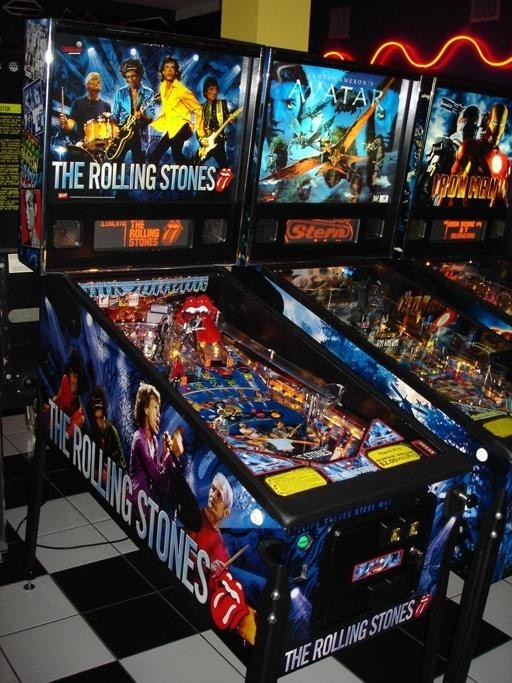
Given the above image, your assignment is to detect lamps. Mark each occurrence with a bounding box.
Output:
[221,1,312,54]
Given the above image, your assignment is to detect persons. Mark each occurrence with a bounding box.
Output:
[87,385,127,489]
[59,71,112,148]
[39,350,86,448]
[99,59,156,196]
[175,471,232,592]
[141,57,203,195]
[124,380,186,534]
[263,62,312,150]
[133,311,331,456]
[194,75,240,195]
